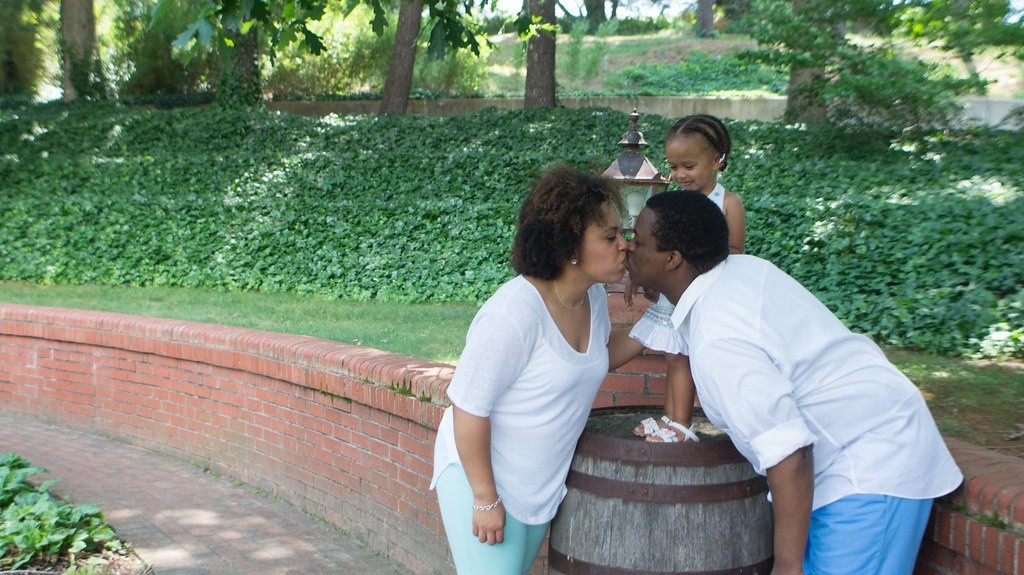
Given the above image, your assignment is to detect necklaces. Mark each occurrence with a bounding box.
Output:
[552,278,584,309]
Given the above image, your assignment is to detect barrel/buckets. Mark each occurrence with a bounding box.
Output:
[548,404,774,575]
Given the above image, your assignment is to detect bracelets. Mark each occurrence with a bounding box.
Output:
[471,495,502,512]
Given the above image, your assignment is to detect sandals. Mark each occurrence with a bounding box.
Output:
[646,421,700,443]
[633,413,671,436]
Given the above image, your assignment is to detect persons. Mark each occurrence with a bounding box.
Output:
[624,115,746,444]
[432,166,647,575]
[624,191,965,575]
